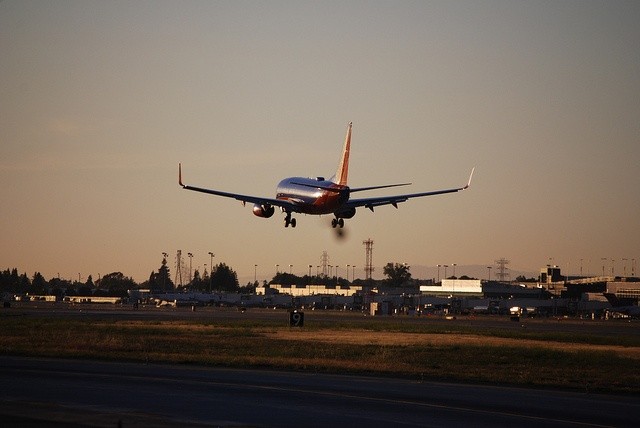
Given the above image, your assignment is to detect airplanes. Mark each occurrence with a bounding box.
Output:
[179,120,475,228]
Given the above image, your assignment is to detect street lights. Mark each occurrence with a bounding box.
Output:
[452,263,456,278]
[437,264,441,283]
[254,264,355,282]
[187,253,194,289]
[208,251,214,291]
[444,264,448,279]
